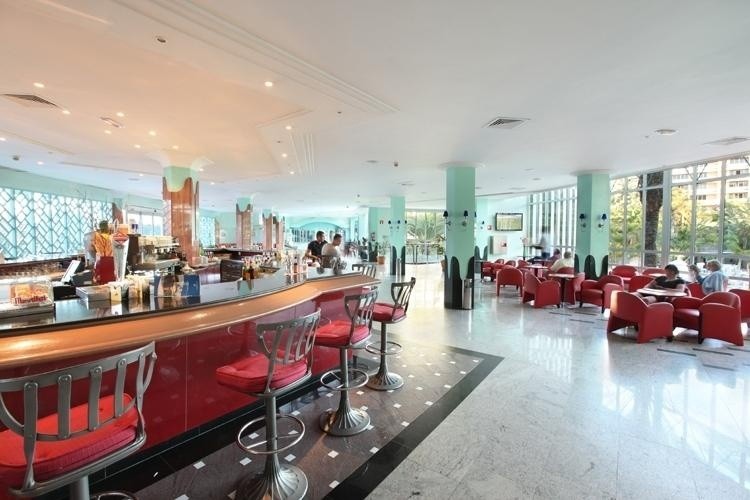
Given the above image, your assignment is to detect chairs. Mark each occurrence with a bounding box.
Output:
[0,342,160,500]
[480,259,750,347]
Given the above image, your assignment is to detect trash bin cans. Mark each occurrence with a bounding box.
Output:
[397,258,401,276]
[463,278,473,310]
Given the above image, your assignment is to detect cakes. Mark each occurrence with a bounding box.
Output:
[10,283,50,307]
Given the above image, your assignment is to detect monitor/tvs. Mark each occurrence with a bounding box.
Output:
[495,212,523,231]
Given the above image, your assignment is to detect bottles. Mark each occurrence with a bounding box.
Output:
[199,250,214,265]
[156,246,176,257]
[245,249,308,281]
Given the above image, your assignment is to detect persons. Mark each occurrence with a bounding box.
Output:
[688,265,704,283]
[531,225,551,258]
[630,264,685,304]
[548,247,561,265]
[699,259,732,296]
[321,233,344,259]
[550,251,574,273]
[306,230,329,261]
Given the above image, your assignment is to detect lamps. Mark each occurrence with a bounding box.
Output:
[442,211,451,226]
[579,213,586,228]
[462,210,469,226]
[598,213,607,228]
